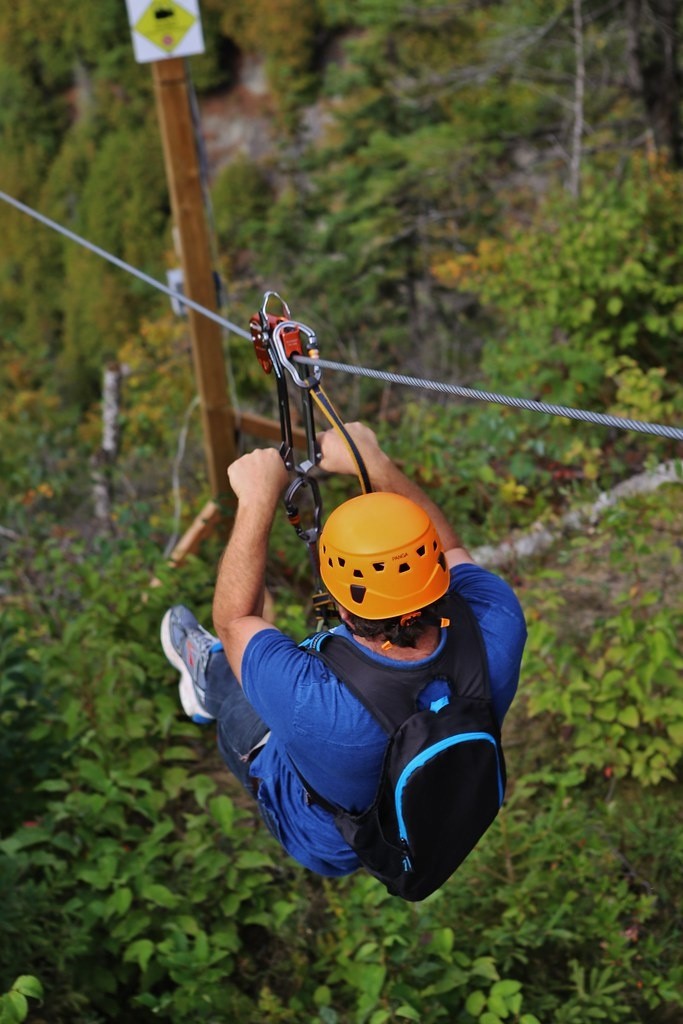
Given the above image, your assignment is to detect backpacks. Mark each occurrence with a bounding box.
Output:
[289,593,508,903]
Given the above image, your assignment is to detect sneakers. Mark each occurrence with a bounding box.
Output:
[161,606,223,725]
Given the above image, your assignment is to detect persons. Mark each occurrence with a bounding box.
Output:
[160,422,528,877]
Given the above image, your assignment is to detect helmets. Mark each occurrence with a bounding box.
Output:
[318,492,450,620]
